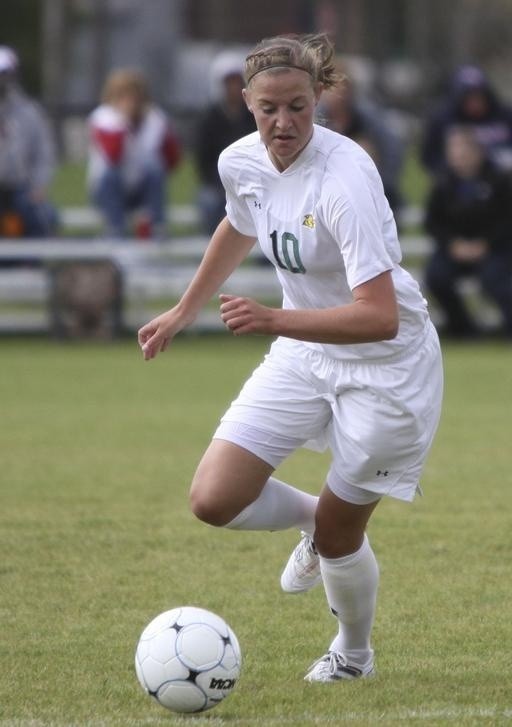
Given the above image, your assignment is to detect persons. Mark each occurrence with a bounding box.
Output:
[86,64,183,244]
[195,46,257,236]
[137,28,445,684]
[1,46,61,268]
[314,56,407,238]
[419,66,511,344]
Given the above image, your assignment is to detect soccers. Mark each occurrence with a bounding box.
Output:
[134,607,242,713]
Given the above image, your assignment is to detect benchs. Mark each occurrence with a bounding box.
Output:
[3,202,501,339]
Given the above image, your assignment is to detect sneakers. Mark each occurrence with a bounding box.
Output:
[304,639,375,685]
[281,534,322,593]
